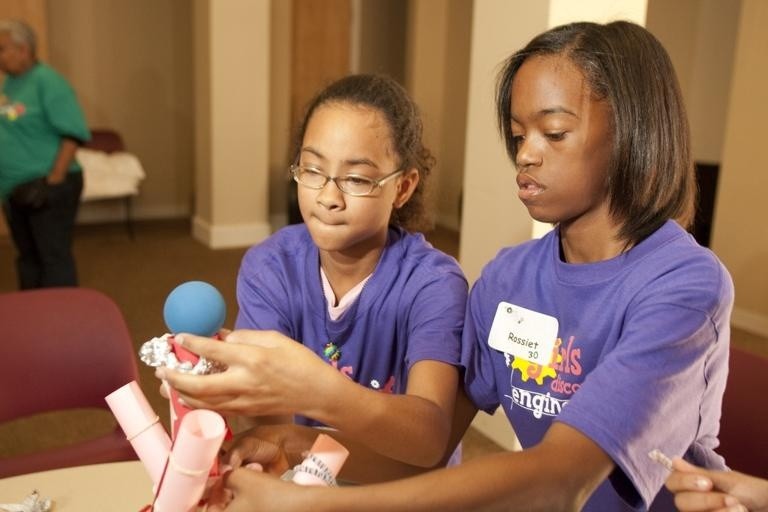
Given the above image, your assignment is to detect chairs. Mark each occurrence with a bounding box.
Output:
[0,286,151,478]
[71,131,134,243]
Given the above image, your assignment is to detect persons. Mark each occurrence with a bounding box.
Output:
[0,19,91,290]
[155,73,468,468]
[205,20,733,512]
[665,455,768,512]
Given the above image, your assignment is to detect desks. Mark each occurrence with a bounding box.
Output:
[5,460,369,512]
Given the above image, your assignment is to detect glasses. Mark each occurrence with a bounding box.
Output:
[290,152,404,196]
